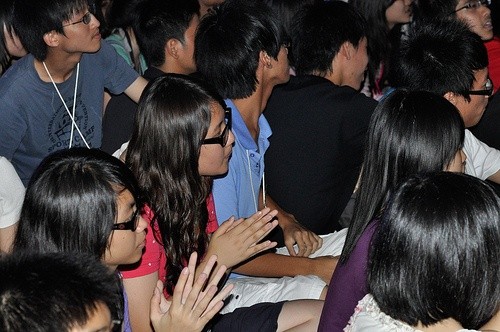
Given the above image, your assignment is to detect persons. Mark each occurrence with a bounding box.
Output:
[260,0,500,332]
[0,0,349,332]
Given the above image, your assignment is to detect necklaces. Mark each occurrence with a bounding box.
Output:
[43,61,90,149]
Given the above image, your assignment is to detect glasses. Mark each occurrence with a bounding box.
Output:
[62,3,96,27]
[205,107,232,148]
[468,77,493,96]
[114,207,142,232]
[456,0,492,12]
